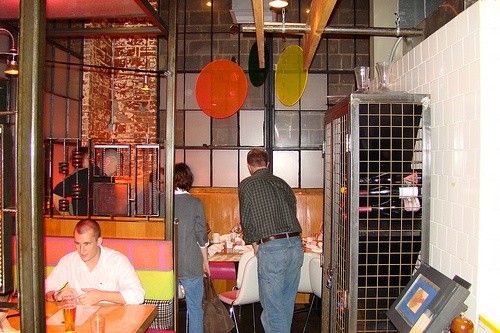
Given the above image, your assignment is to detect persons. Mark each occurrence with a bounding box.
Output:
[120,167,164,215]
[160,162,211,333]
[45,219,145,305]
[238,148,304,333]
[53,147,123,216]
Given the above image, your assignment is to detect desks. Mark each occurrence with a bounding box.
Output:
[209,252,242,262]
[0,298,159,333]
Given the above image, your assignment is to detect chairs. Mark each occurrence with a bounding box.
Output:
[290,252,323,333]
[219,251,260,333]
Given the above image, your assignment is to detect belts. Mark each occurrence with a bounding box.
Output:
[257,232,300,245]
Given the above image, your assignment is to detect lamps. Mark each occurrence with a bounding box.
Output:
[268,0,289,8]
[0,28,19,75]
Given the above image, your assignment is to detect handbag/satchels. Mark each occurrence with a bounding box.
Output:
[203,271,235,333]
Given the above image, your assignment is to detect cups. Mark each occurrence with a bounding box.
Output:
[62,295,77,333]
[354,66,371,94]
[89,312,106,333]
[374,62,393,93]
[230,233,237,242]
[211,232,220,243]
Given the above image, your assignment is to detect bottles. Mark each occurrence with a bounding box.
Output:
[337,171,422,220]
[450,311,474,333]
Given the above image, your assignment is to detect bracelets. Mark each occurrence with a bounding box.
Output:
[52,294,56,300]
